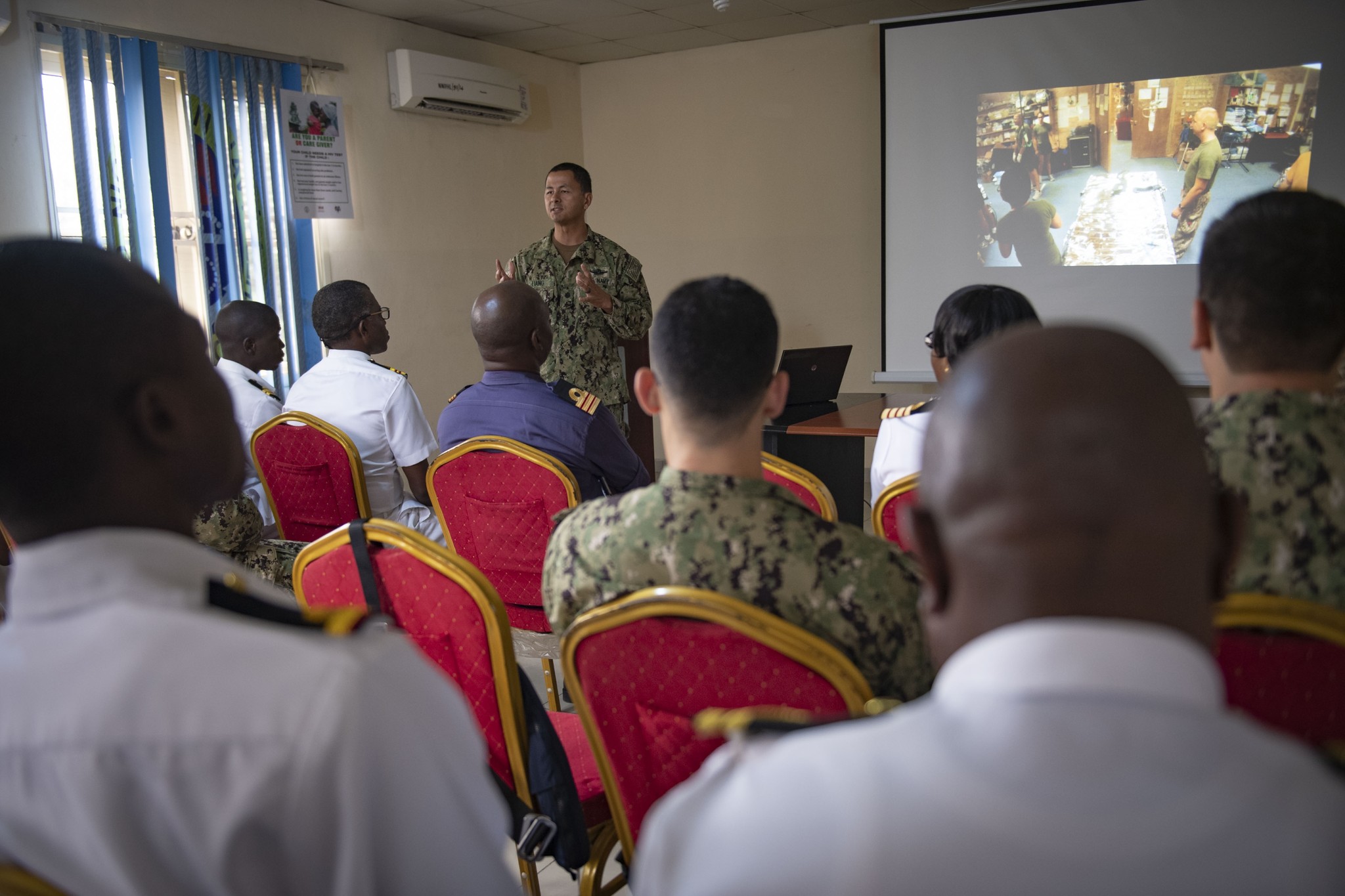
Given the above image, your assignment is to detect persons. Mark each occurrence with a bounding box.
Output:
[0,239,522,896]
[629,331,1345,896]
[495,163,653,440]
[975,111,1062,267]
[1171,107,1222,263]
[215,188,1345,700]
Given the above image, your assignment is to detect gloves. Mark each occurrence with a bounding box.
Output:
[1013,152,1022,163]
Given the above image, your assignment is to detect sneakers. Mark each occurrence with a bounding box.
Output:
[1049,175,1055,181]
[992,232,997,240]
[979,237,994,248]
[1039,177,1042,183]
[1032,185,1036,191]
[1032,190,1042,200]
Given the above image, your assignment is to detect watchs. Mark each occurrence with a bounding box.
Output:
[1179,203,1185,210]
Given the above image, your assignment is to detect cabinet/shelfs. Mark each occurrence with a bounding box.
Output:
[975,97,1056,148]
[1217,84,1264,126]
[1069,136,1092,168]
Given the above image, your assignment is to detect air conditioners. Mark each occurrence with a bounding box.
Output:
[386,48,530,124]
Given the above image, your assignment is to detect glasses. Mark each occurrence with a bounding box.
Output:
[997,186,1003,192]
[367,307,390,320]
[924,331,934,348]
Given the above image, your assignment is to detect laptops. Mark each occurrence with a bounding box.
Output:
[777,344,853,405]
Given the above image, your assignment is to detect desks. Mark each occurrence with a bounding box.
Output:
[1246,131,1289,164]
[753,392,938,533]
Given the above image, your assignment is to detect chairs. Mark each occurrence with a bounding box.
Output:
[248,411,1345,895]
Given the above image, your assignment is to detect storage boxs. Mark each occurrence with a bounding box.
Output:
[1177,141,1249,171]
[982,135,1017,183]
